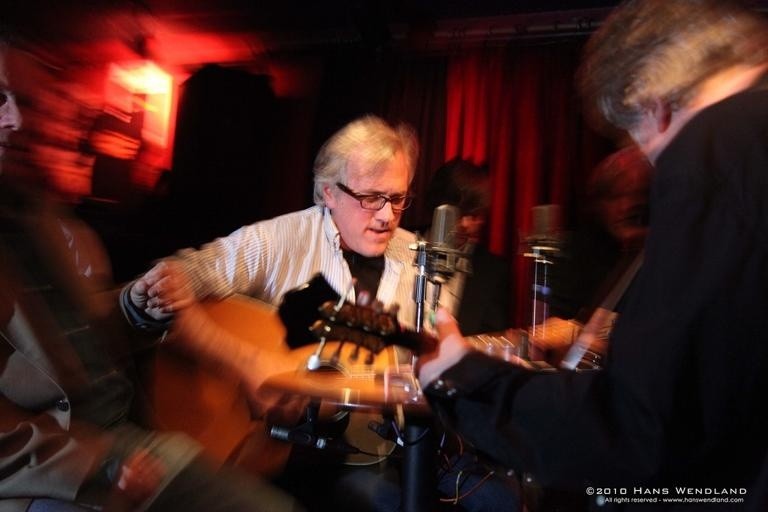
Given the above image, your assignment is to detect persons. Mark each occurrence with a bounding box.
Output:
[1,0,768,512]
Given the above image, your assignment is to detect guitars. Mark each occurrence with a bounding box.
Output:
[138,293,581,479]
[276,272,608,511]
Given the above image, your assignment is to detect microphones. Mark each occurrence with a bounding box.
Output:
[524,202,563,265]
[426,202,473,284]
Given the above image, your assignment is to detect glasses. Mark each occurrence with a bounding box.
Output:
[336,182,412,212]
